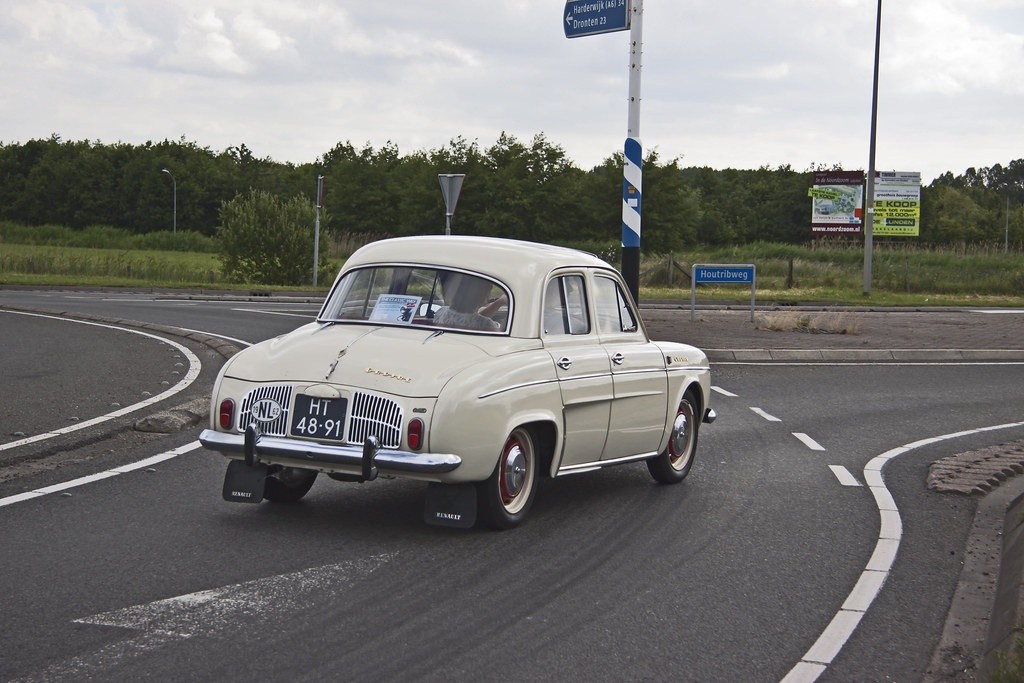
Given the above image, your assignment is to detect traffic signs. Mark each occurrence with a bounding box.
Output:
[563,0,631,38]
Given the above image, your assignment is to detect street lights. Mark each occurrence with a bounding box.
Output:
[162,168,177,233]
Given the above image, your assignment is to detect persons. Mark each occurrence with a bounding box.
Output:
[542,277,586,335]
[433,269,509,332]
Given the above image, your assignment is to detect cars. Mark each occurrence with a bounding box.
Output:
[200,235,716,535]
